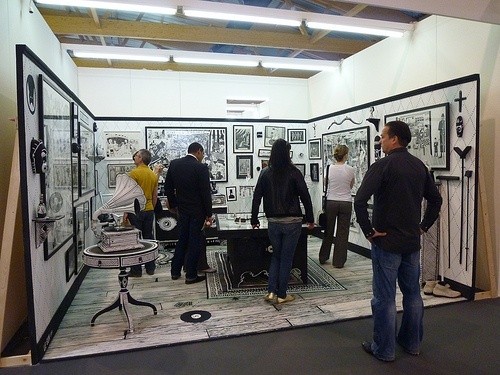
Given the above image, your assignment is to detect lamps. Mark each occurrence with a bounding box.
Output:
[29,0,414,72]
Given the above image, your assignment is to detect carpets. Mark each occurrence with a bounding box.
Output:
[205,246,347,296]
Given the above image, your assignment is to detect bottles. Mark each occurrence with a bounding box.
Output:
[38,194,46,218]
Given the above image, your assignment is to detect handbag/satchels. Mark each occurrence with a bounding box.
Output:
[319,213,327,226]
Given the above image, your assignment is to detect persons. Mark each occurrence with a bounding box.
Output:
[318,145,355,269]
[123,142,217,284]
[354,121,443,363]
[250,138,315,303]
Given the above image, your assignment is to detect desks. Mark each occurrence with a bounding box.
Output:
[82,241,160,332]
[216,213,321,285]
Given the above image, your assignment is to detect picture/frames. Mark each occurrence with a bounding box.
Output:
[383,102,450,169]
[309,163,318,181]
[287,128,306,144]
[294,163,306,177]
[103,130,143,160]
[239,184,254,198]
[321,125,372,198]
[108,163,139,190]
[264,126,286,147]
[350,210,360,232]
[236,155,253,178]
[38,74,99,282]
[226,187,237,201]
[232,125,255,153]
[308,138,321,160]
[145,126,228,181]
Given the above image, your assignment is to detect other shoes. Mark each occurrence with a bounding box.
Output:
[171,272,182,280]
[185,276,206,285]
[127,269,142,277]
[197,268,217,274]
[362,338,376,357]
[263,292,274,300]
[145,269,155,275]
[278,295,295,303]
